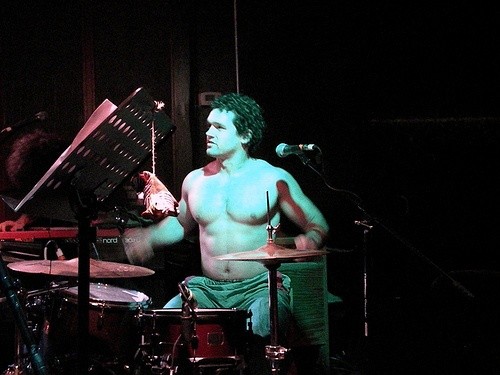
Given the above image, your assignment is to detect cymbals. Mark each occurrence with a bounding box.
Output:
[7,257,155,279]
[211,244,331,265]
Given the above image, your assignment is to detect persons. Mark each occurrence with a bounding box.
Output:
[0,129,62,232]
[123,92,330,337]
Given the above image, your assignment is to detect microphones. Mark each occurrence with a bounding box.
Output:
[276,143,317,158]
[0,111,49,135]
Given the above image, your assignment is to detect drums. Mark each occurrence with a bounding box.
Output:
[134,309,252,369]
[46,282,153,375]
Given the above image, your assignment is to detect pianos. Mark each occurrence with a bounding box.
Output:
[0,227,121,248]
